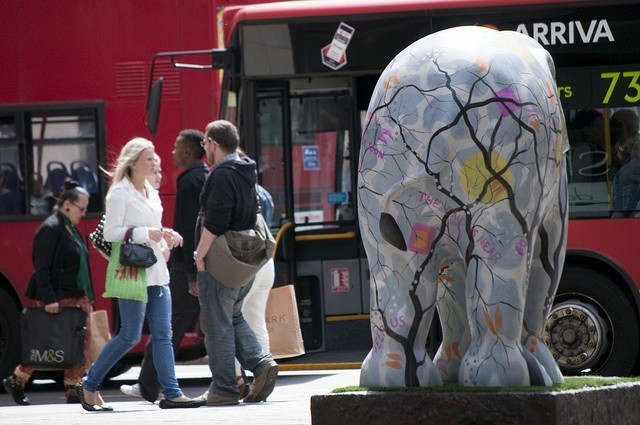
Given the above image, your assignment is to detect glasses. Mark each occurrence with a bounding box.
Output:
[70,201,87,213]
[200,139,221,148]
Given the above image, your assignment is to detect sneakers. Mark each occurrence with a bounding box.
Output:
[158,397,206,409]
[79,386,103,411]
[3,374,31,405]
[193,389,239,406]
[120,383,164,399]
[243,360,279,402]
[234,375,250,401]
[63,384,81,403]
[100,403,114,411]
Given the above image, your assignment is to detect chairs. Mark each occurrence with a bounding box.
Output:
[47,160,70,194]
[0,162,24,217]
[335,202,356,222]
[71,160,99,194]
[571,142,606,182]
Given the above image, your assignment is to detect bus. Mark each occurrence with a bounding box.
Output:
[146,1,640,376]
[1,1,384,395]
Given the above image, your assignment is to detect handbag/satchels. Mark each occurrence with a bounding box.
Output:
[194,211,276,288]
[119,228,157,267]
[88,214,112,261]
[18,307,87,370]
[264,284,307,360]
[101,242,149,303]
[83,309,111,367]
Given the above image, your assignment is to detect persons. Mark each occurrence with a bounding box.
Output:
[120,131,211,402]
[74,137,206,412]
[3,178,95,405]
[222,149,276,398]
[189,120,278,406]
[88,153,170,411]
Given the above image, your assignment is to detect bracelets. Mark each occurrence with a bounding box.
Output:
[191,251,207,261]
